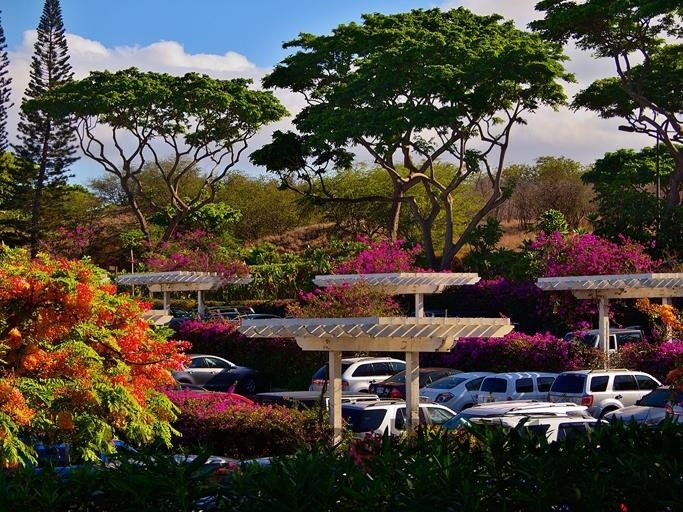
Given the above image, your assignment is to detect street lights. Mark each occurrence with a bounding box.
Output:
[615,124,662,234]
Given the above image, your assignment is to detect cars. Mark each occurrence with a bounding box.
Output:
[166,381,206,393]
[169,353,269,394]
[154,302,285,322]
[15,437,296,509]
[564,322,646,354]
